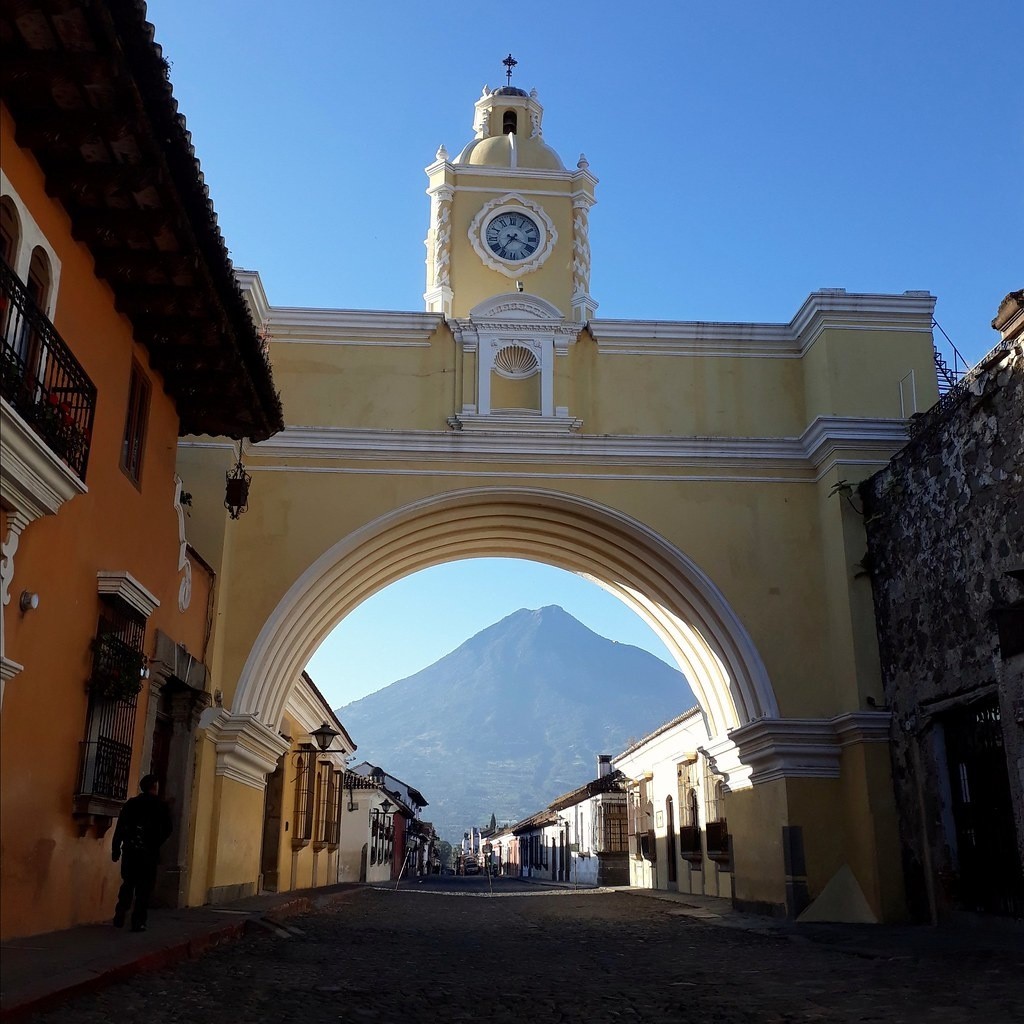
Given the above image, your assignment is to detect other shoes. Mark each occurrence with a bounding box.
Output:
[113,907,125,928]
[131,920,147,932]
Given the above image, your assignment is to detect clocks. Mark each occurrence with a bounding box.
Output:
[480,204,547,265]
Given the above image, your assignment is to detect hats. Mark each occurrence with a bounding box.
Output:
[140,775,161,791]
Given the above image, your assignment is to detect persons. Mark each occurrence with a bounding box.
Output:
[112,775,172,931]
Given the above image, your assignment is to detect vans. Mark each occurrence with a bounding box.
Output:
[465,862,479,874]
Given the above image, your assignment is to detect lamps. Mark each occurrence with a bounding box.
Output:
[290,721,346,783]
[369,798,395,829]
[828,479,863,515]
[224,440,251,520]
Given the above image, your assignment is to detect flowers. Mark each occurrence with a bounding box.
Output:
[0,352,91,468]
